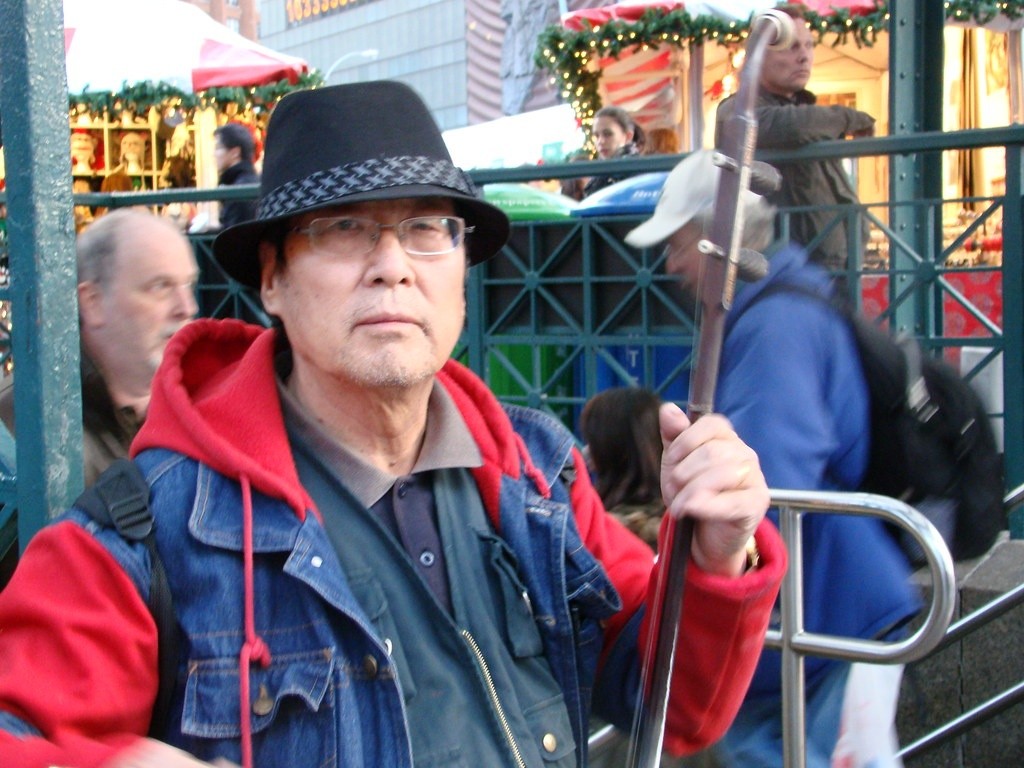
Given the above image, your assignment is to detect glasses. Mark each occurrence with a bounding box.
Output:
[287,216,477,258]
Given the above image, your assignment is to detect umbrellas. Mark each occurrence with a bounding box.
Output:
[65,1,315,94]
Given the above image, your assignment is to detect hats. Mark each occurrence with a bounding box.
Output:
[211,79,511,288]
[623,149,761,248]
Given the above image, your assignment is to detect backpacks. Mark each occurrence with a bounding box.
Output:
[741,273,1004,575]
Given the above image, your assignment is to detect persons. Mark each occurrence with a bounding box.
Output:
[712,6,877,317]
[0,206,204,595]
[1,77,791,767]
[156,155,196,231]
[579,108,649,198]
[626,143,920,767]
[211,122,266,230]
[98,173,134,197]
[576,387,738,768]
